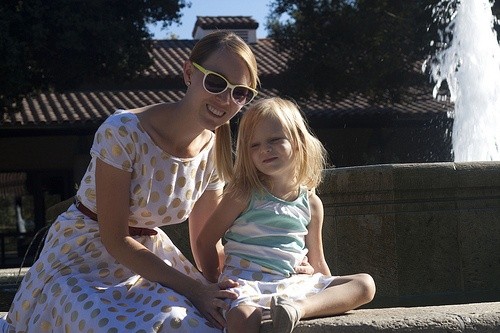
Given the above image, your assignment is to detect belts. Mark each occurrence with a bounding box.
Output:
[74,196,158,236]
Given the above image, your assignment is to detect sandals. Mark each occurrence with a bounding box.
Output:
[271,294,299,333]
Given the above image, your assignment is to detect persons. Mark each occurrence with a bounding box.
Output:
[197,97,376,333]
[2,31,259,332]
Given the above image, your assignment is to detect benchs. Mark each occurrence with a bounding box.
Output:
[46,163,500,333]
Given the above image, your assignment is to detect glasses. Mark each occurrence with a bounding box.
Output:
[193,62,258,106]
